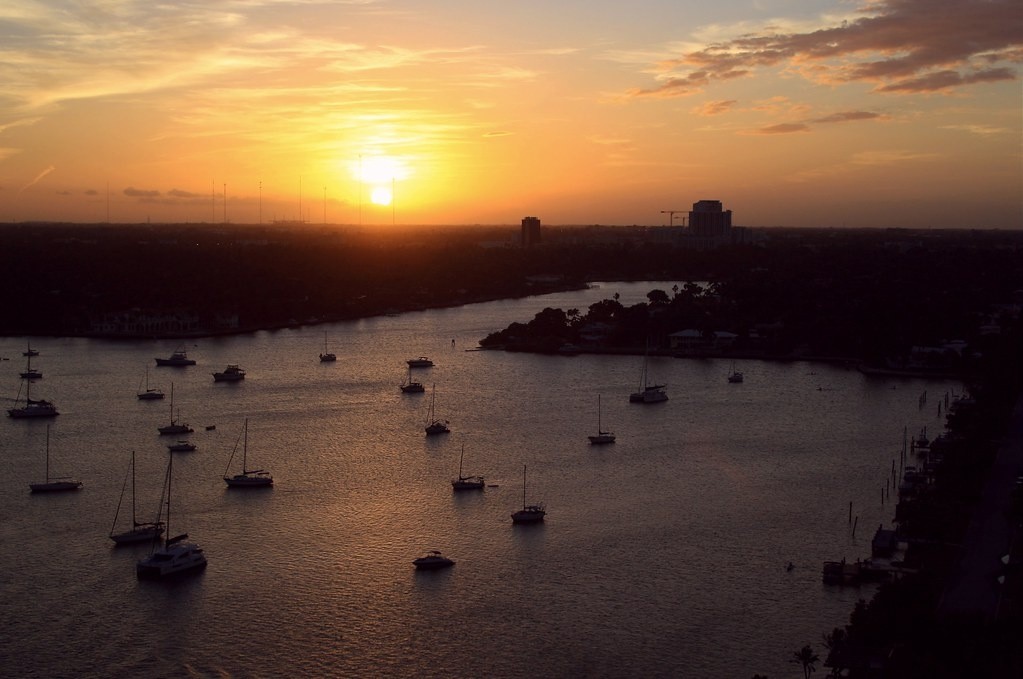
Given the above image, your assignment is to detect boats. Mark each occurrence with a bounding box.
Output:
[152,340,197,367]
[165,439,197,452]
[211,363,247,382]
[22,351,40,356]
[18,372,43,379]
[406,357,435,368]
[726,359,745,383]
[411,549,457,571]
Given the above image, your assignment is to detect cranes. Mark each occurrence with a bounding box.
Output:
[659,210,690,228]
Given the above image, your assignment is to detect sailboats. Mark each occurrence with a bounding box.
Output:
[5,340,61,420]
[318,331,337,361]
[109,448,166,547]
[588,395,616,444]
[136,451,208,585]
[157,382,195,436]
[510,465,547,523]
[451,440,486,491]
[27,424,84,493]
[630,332,669,403]
[400,366,426,393]
[136,363,165,399]
[222,417,275,489]
